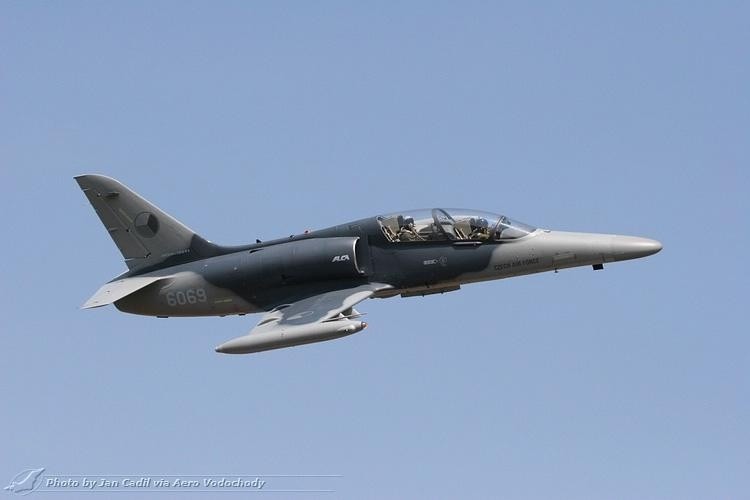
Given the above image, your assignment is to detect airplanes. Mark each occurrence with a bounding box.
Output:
[70,171,665,357]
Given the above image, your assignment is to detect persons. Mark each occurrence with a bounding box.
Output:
[400,216,420,241]
[472,218,490,239]
